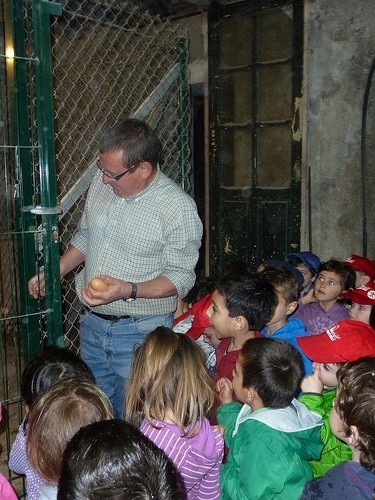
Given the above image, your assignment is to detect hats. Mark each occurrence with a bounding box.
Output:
[296,321,375,363]
[342,255,375,274]
[286,251,320,270]
[341,283,375,305]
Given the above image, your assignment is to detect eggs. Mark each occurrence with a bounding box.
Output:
[86,277,108,299]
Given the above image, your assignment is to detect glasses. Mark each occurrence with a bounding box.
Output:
[96,158,139,180]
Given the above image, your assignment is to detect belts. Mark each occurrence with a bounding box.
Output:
[84,305,130,320]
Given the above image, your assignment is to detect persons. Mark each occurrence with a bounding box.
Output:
[0,251,375,500]
[28,118,203,421]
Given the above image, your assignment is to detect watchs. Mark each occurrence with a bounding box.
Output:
[123,282,136,303]
